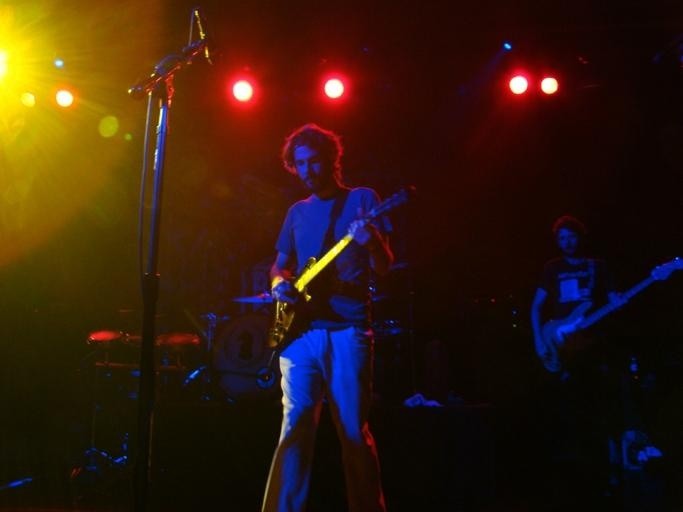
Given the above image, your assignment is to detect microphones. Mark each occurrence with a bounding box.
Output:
[194,10,220,66]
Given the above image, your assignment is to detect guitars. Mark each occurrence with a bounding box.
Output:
[267,183,418,352]
[538,255,683,372]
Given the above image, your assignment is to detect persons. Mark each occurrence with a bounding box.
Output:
[259,123,397,511]
[528,212,629,375]
